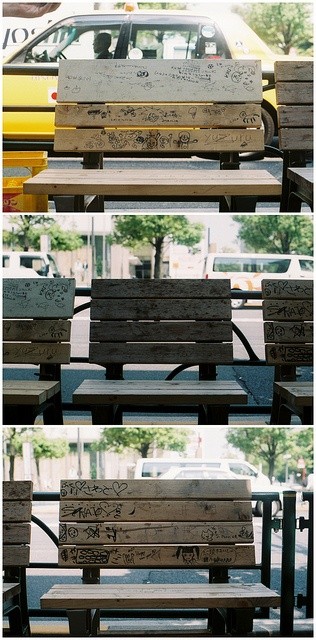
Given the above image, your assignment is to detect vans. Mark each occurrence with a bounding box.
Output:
[203,254,312,310]
[133,460,267,486]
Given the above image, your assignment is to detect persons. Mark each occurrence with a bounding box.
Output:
[3,3,61,18]
[92,33,113,59]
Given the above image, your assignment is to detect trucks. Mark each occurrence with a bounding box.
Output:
[3,252,60,278]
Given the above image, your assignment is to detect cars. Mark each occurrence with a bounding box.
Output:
[2,1,314,162]
[159,466,295,517]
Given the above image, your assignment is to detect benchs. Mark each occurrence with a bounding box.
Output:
[23,59,282,212]
[3,277,76,425]
[261,279,313,425]
[274,60,313,212]
[39,479,281,637]
[3,151,49,212]
[3,481,33,636]
[72,279,248,425]
